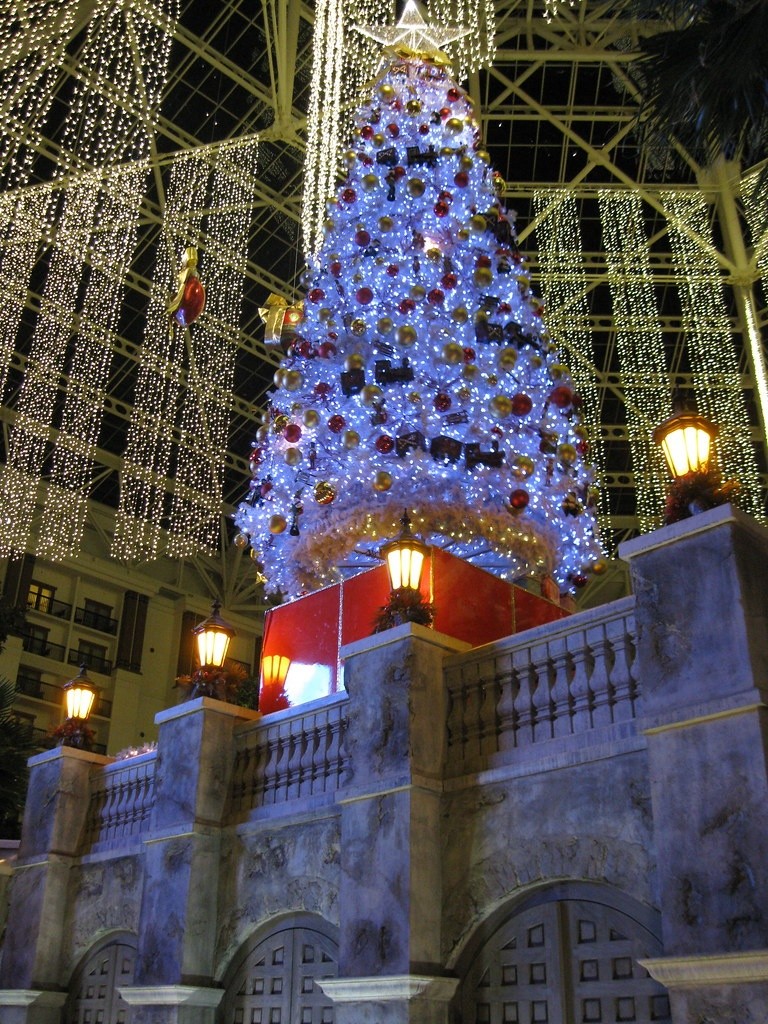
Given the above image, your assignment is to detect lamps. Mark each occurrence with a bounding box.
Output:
[59,657,98,752]
[191,594,236,701]
[652,383,718,515]
[262,634,291,715]
[379,508,431,627]
